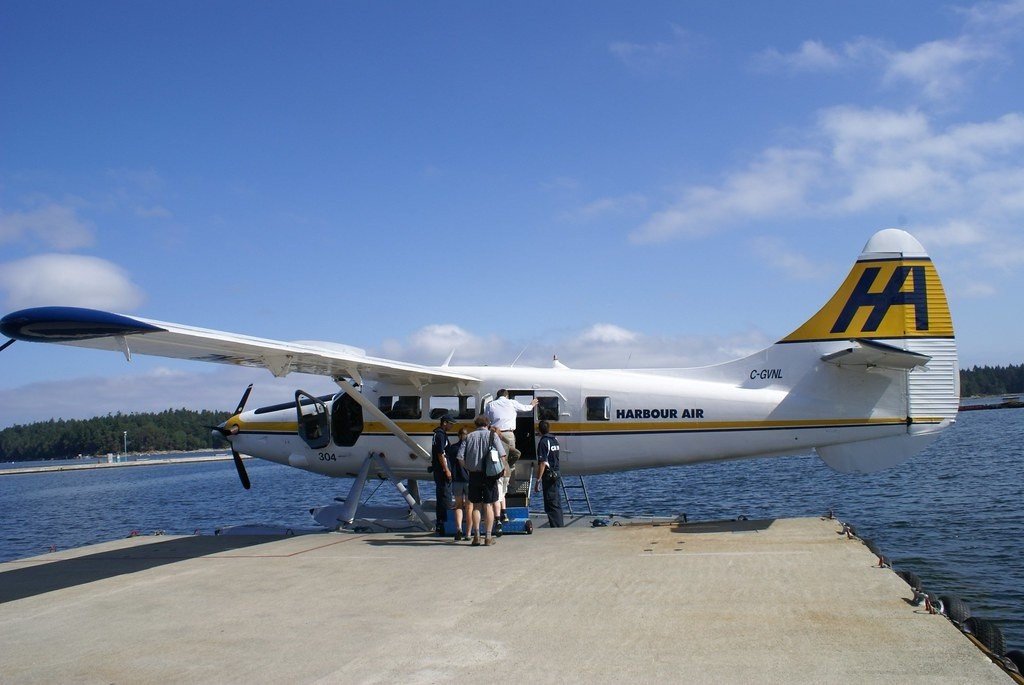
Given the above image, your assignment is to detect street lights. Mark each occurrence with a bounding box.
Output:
[123,431,127,462]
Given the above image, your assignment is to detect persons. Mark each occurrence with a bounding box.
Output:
[451,416,521,546]
[432,415,456,535]
[535,421,564,527]
[486,389,539,494]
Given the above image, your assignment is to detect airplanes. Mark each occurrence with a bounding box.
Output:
[0,227,961,536]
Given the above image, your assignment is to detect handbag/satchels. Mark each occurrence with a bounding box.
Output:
[486,430,505,480]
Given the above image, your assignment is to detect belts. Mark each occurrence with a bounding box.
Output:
[501,430,513,432]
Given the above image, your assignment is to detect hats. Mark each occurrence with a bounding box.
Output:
[440,414,457,424]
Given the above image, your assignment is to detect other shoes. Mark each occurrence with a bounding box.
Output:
[472,537,481,546]
[485,538,496,545]
[503,514,509,522]
[496,522,502,537]
[455,530,462,541]
[465,536,472,540]
[436,523,441,533]
